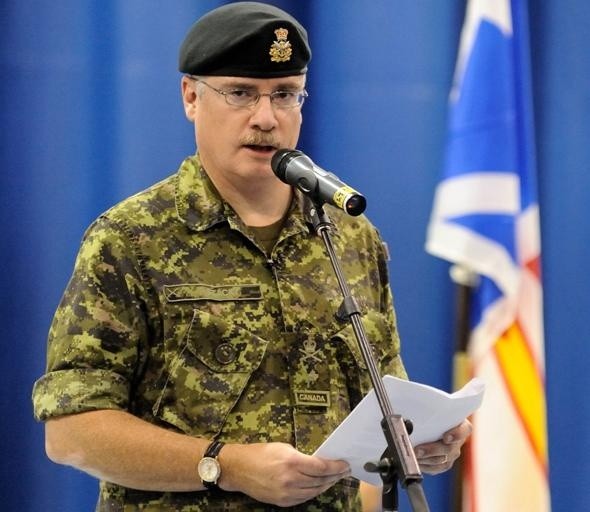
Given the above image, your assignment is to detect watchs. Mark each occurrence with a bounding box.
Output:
[197,441,226,499]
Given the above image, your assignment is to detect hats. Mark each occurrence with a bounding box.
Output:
[179,1,311,75]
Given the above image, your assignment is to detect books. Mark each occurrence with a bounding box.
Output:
[311,375,485,488]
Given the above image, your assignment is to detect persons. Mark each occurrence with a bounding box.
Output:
[32,2,473,511]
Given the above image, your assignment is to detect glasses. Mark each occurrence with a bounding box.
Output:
[191,76,308,108]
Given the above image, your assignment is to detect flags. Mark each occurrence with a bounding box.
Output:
[424,0,553,512]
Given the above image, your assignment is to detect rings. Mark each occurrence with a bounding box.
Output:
[445,455,448,463]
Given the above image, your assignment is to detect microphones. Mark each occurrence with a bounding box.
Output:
[271,147,366,217]
